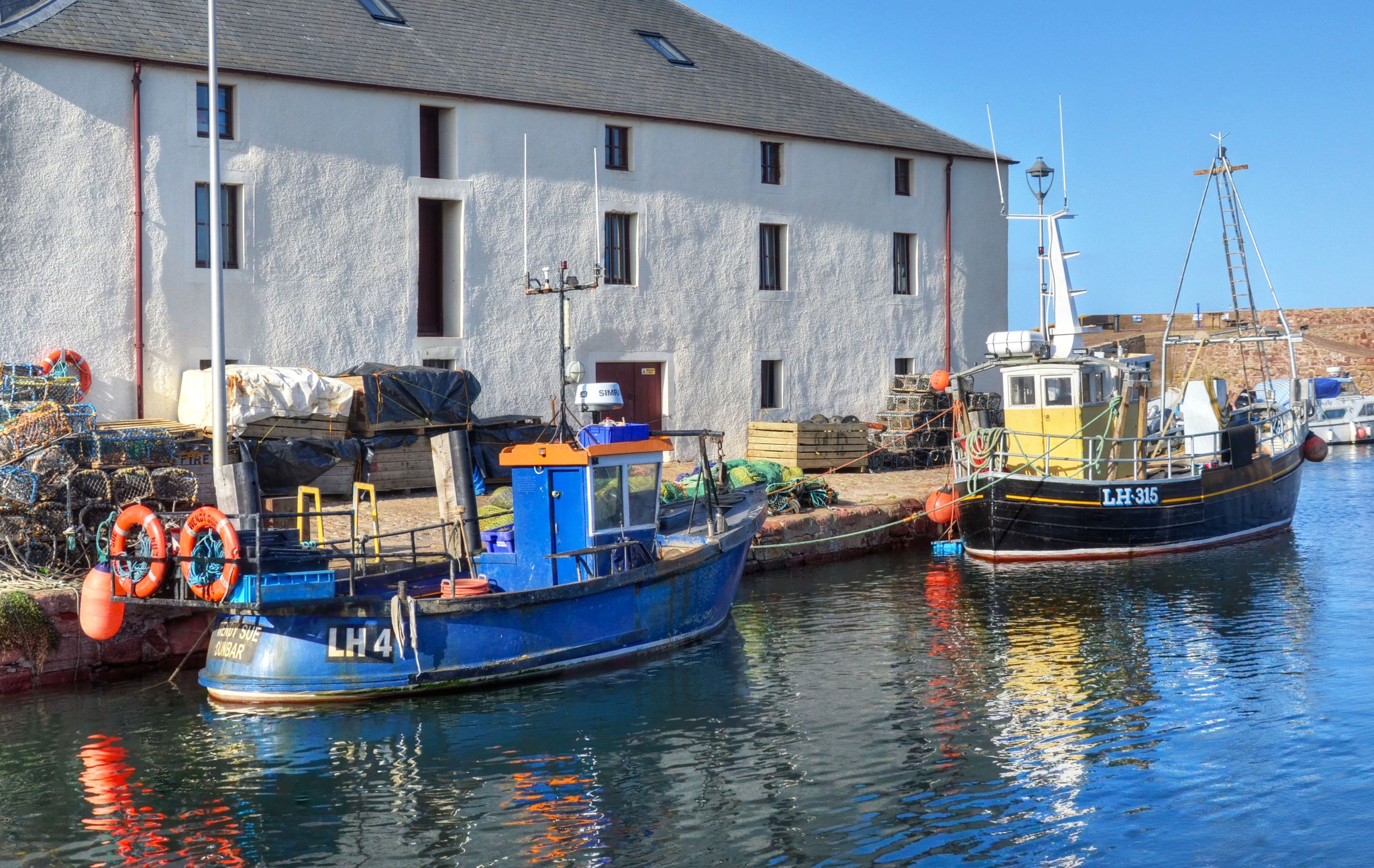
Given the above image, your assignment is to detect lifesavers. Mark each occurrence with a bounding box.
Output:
[109,505,167,595]
[177,506,240,601]
[43,347,92,402]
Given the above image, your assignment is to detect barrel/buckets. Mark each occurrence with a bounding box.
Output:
[440,574,490,599]
[1194,460,1218,475]
[170,527,181,555]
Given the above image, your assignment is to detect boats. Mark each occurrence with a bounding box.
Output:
[1254,365,1374,445]
[949,92,1316,560]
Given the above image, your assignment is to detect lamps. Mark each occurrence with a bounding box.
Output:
[566,359,586,385]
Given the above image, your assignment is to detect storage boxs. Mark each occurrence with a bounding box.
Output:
[747,421,869,473]
[205,528,337,604]
[480,523,515,553]
[240,376,544,495]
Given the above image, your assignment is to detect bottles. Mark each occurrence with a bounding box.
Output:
[604,417,613,427]
[1117,345,1122,359]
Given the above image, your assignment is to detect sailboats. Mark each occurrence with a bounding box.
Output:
[109,0,771,706]
